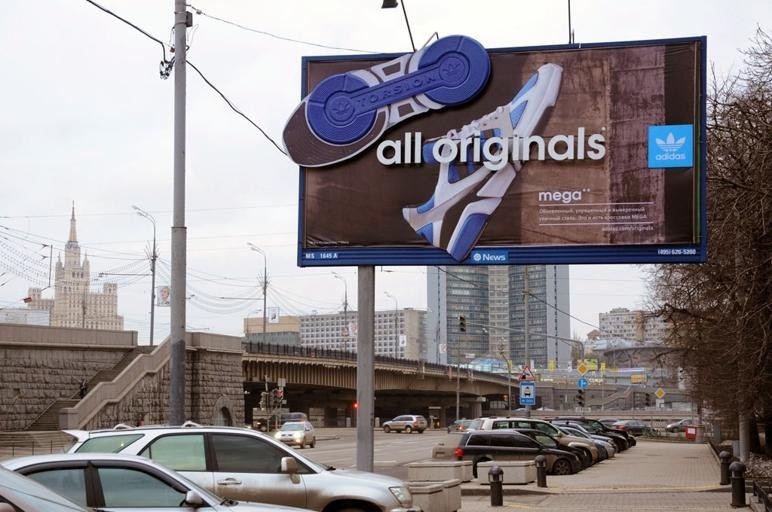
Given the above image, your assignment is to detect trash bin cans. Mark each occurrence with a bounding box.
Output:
[695,425,705,444]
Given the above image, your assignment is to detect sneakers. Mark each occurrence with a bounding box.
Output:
[400,61,565,266]
[280,32,494,170]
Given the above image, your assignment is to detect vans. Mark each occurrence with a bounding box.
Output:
[256,411,308,432]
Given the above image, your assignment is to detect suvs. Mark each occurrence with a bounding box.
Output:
[273,420,317,449]
[382,414,428,434]
[53,418,412,511]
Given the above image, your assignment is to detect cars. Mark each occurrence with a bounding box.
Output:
[431,414,651,476]
[664,418,713,433]
[1,452,318,510]
[1,464,78,511]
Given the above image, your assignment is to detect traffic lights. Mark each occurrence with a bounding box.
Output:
[270,388,284,408]
[644,392,650,407]
[354,401,358,410]
[511,393,515,406]
[576,388,584,407]
[458,315,466,333]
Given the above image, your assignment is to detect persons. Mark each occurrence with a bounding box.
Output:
[79,377,89,398]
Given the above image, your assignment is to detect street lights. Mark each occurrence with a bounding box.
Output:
[325,270,349,360]
[244,239,271,342]
[129,202,159,346]
[480,327,488,355]
[381,289,400,361]
